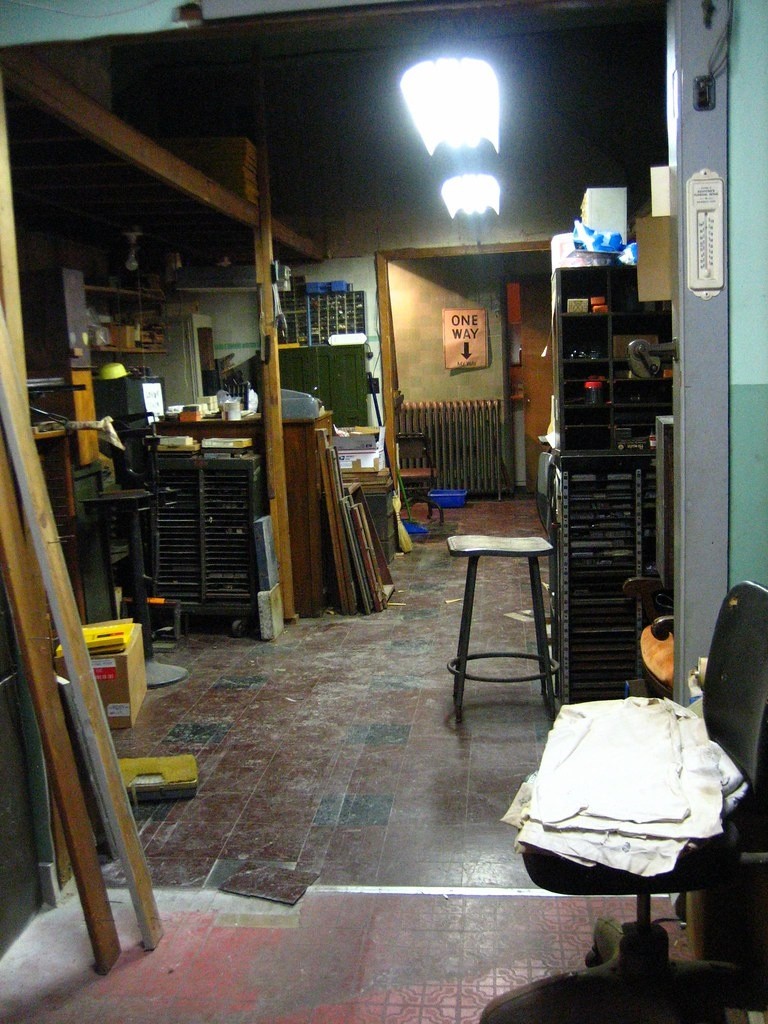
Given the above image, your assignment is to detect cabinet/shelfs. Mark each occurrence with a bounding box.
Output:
[83,285,168,353]
[255,344,373,428]
[279,291,368,345]
[550,265,674,455]
[151,409,333,618]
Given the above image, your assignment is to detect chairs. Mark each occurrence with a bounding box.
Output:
[477,960,752,1024]
[395,431,446,527]
[519,581,767,967]
[623,576,674,697]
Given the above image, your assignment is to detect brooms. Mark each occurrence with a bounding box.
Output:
[367,371,428,533]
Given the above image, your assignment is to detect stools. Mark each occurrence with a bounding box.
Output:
[445,533,560,720]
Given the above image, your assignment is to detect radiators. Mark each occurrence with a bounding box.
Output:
[399,398,511,502]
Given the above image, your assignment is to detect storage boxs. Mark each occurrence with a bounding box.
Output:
[54,630,148,730]
[32,41,112,111]
[550,231,605,270]
[580,188,628,245]
[613,335,659,358]
[567,299,588,313]
[160,135,259,205]
[427,488,468,508]
[331,425,387,473]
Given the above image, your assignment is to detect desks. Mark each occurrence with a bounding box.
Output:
[79,487,186,690]
